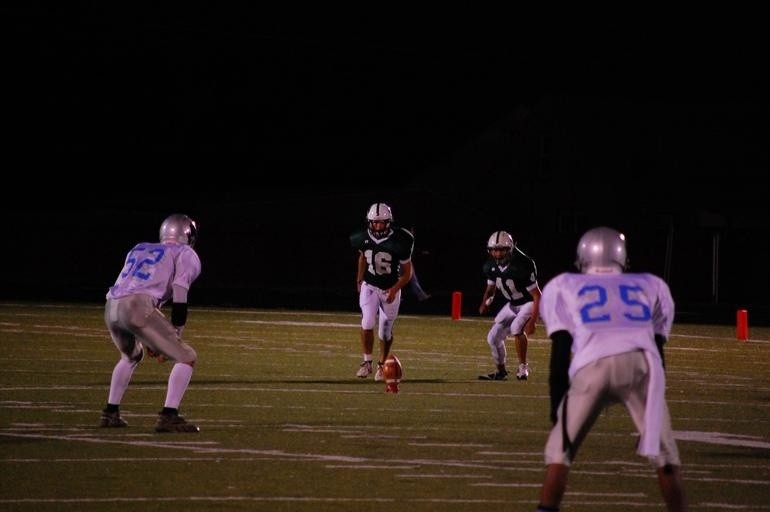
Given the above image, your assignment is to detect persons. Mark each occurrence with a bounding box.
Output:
[353,202,418,381]
[98,213,200,431]
[476,231,545,381]
[533,228,693,511]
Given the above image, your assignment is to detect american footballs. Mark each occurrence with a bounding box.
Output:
[383,353,402,385]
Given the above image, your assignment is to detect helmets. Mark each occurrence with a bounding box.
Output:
[366,203,394,238]
[159,213,199,249]
[487,230,514,255]
[577,226,628,274]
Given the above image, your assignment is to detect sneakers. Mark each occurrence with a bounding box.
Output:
[516,367,529,381]
[478,371,508,380]
[99,410,128,428]
[374,363,385,382]
[155,414,200,432]
[356,360,372,377]
